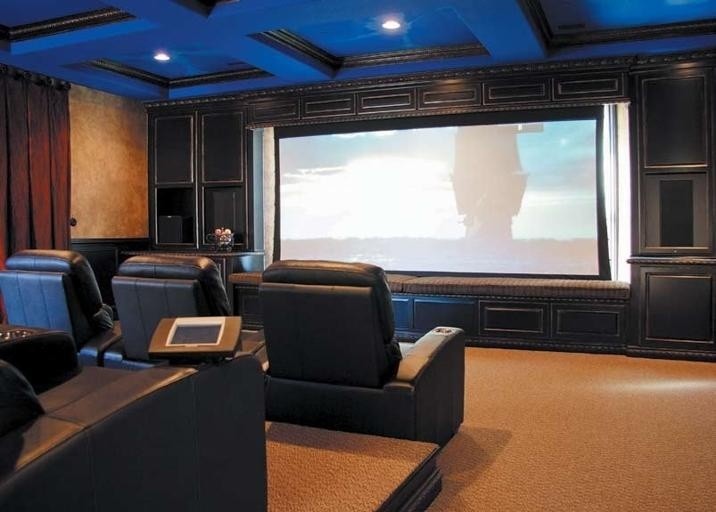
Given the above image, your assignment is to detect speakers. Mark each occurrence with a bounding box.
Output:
[158,215,193,243]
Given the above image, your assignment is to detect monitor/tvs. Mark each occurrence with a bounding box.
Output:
[147,317,243,356]
